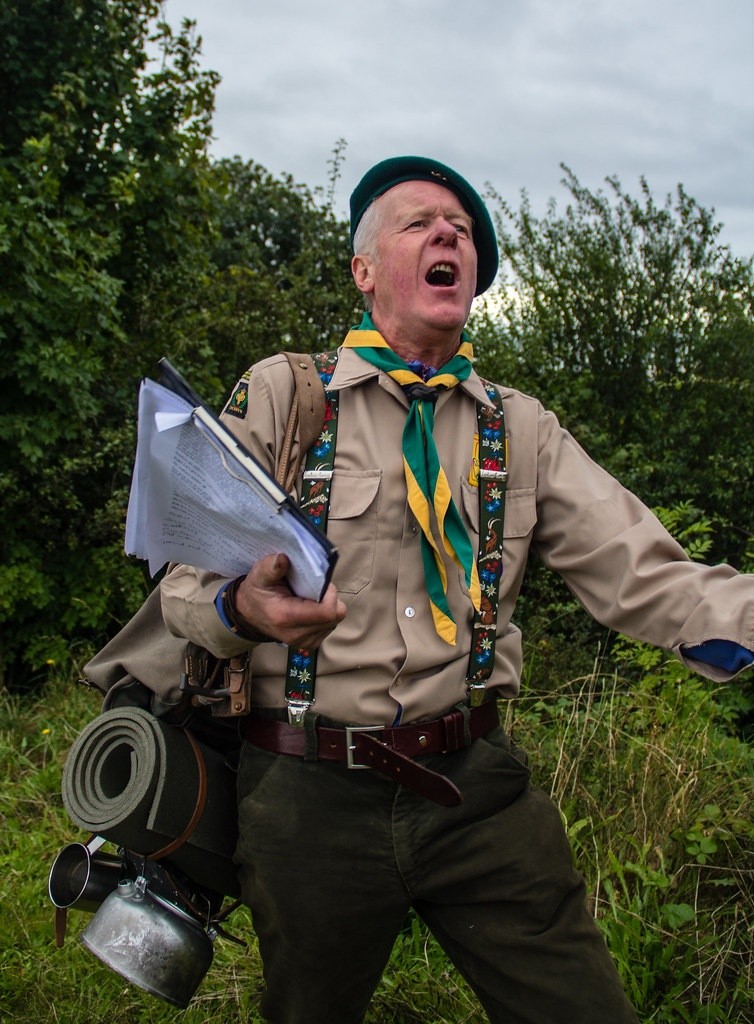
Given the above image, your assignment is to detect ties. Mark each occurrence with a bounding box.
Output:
[344,312,482,647]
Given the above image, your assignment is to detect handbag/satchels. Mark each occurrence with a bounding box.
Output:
[82,561,251,720]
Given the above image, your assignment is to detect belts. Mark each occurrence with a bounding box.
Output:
[230,697,504,809]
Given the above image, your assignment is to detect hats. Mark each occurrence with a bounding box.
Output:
[349,156,499,299]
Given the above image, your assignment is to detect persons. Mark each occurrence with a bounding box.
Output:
[160,156,754,1024]
